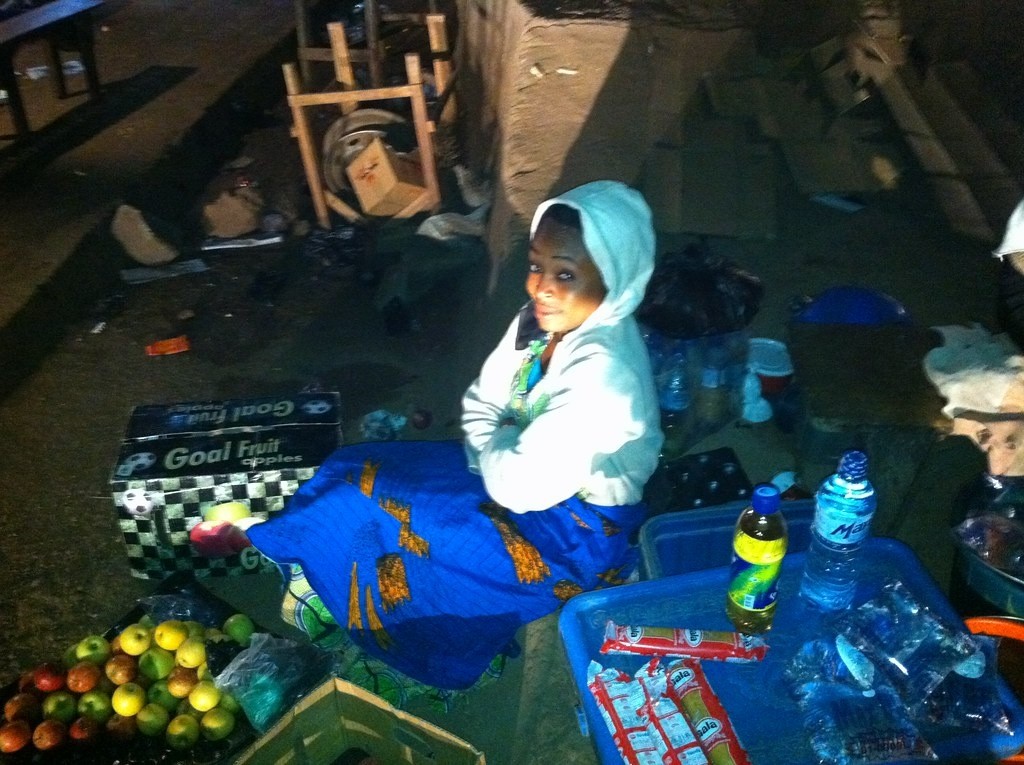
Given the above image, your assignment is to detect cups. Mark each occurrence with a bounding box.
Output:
[736,337,798,426]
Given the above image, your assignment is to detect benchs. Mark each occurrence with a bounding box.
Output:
[0,0,107,140]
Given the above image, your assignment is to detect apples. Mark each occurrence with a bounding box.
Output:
[0,614,255,759]
[190,500,255,558]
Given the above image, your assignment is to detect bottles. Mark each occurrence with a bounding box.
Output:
[642,333,747,448]
[796,451,880,622]
[724,483,789,634]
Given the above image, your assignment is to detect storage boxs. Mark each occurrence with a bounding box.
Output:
[237,677,486,765]
[556,497,1024,765]
[114,391,344,600]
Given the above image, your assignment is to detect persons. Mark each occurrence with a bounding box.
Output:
[243,179,664,694]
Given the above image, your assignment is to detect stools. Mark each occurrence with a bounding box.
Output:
[281,0,458,227]
